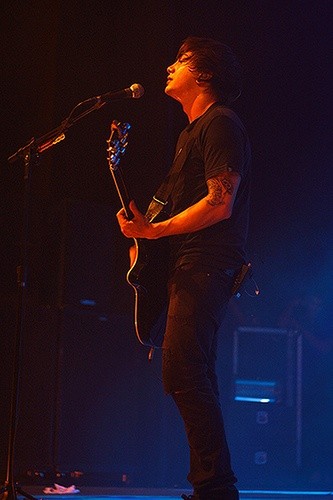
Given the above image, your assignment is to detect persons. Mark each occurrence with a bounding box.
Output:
[115,36,248,500]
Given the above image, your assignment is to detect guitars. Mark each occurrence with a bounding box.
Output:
[107,120,173,349]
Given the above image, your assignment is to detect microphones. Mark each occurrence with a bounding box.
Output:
[80,83,144,105]
